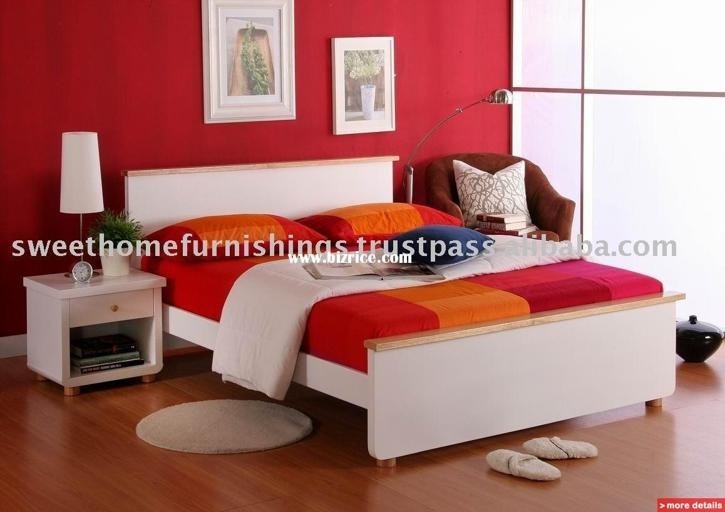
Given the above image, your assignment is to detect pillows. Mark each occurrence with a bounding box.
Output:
[452,159,539,232]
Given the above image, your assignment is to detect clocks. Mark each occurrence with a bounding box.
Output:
[72,261,93,285]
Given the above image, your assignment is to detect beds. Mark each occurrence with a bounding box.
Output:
[121,155,687,467]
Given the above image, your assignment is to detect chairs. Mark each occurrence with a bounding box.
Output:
[422,152,575,242]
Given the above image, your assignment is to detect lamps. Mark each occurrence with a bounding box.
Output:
[402,88,514,203]
[59,130,105,278]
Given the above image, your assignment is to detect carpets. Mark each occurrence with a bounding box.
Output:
[136,399,316,457]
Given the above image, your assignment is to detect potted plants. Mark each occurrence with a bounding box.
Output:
[84,209,145,277]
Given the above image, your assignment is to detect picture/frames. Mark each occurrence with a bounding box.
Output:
[200,0,297,126]
[331,35,396,136]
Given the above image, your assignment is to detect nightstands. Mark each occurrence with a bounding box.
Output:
[23,266,167,396]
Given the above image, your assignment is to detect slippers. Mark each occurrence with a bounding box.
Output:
[487,447,561,480]
[522,436,600,462]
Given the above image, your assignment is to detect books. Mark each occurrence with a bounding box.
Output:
[476,220,526,231]
[476,225,538,236]
[70,358,145,375]
[303,260,446,281]
[71,333,137,358]
[477,212,527,224]
[71,351,140,367]
